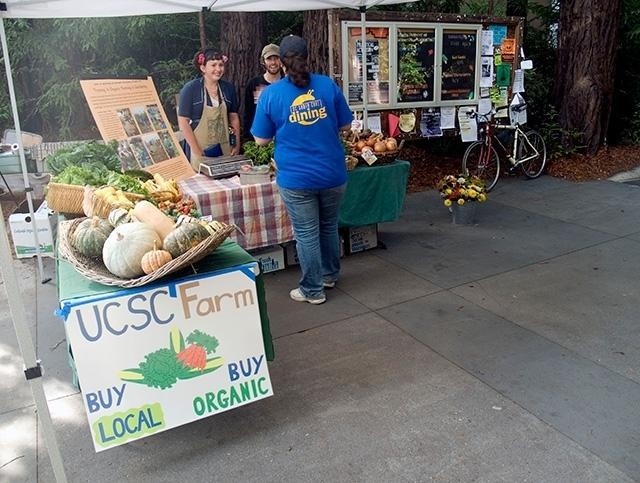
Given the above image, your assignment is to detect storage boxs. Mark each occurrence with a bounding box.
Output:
[248,222,380,274]
[8,198,57,259]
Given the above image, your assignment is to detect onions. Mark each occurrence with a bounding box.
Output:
[341,129,397,153]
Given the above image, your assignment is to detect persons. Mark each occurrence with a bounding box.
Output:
[178,47,240,164]
[240,43,285,155]
[249,35,352,304]
[117,105,179,171]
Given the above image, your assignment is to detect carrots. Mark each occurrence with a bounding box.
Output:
[177,344,206,373]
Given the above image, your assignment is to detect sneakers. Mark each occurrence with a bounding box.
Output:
[290,282,335,304]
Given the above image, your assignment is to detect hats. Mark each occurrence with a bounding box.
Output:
[280,34,307,62]
[261,44,280,59]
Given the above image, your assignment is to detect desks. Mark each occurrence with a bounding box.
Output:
[177,159,411,249]
[0,147,36,208]
[56,217,275,394]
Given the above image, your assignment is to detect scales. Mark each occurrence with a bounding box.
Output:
[199,155,254,177]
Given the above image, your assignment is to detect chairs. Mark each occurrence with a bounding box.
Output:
[2,128,44,152]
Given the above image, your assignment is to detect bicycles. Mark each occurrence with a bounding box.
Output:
[462,101,547,192]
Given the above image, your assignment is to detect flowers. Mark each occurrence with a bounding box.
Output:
[439,174,487,211]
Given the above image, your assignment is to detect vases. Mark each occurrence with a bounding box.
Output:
[450,201,478,225]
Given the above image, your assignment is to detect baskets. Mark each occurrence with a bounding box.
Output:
[344,139,406,171]
[47,181,183,214]
[56,217,237,289]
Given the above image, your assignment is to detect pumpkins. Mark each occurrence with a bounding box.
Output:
[75,216,110,256]
[133,201,173,240]
[162,223,214,258]
[107,208,140,231]
[141,241,171,273]
[102,223,163,277]
